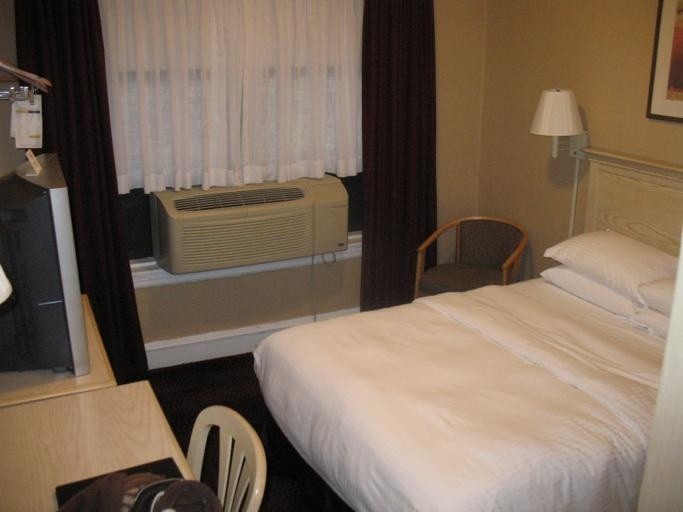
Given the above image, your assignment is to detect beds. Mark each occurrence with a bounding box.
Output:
[251,148,683,503]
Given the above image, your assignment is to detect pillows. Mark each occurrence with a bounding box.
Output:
[541,224,675,335]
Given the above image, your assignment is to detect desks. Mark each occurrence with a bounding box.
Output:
[0,293,201,512]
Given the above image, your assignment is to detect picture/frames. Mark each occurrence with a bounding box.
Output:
[644,0,683,122]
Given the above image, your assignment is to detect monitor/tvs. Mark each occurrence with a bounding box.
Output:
[0,153,92,377]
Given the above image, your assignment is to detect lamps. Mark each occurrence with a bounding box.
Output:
[530,84,592,164]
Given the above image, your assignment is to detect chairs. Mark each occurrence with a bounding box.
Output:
[184,401,268,512]
[414,214,530,298]
